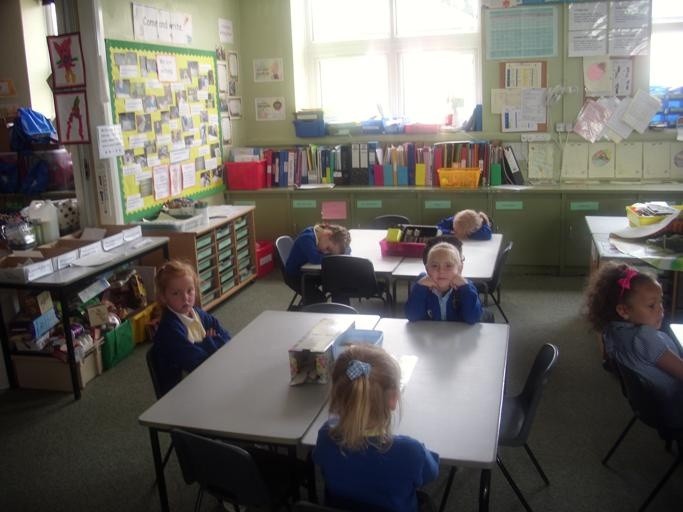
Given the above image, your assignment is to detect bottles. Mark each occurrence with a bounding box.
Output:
[32,218,44,246]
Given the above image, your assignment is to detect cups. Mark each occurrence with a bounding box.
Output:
[195,207,210,224]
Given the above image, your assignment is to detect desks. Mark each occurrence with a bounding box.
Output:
[0,234,172,400]
[140,310,383,508]
[392,230,511,324]
[301,311,513,508]
[345,227,406,316]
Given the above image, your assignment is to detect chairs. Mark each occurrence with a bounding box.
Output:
[366,213,412,230]
[439,338,561,510]
[316,254,394,317]
[146,341,176,402]
[296,482,440,511]
[271,234,327,311]
[171,427,313,512]
[474,240,516,323]
[299,300,360,316]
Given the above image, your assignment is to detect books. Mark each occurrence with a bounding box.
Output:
[232,141,524,187]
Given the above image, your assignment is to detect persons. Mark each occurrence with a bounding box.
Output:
[153,259,231,397]
[283,223,352,306]
[313,343,440,512]
[405,241,482,325]
[582,261,683,396]
[436,209,492,240]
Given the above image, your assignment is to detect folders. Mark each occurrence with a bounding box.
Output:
[504,146,524,186]
[348,142,369,185]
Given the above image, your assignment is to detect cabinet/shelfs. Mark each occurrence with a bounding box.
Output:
[422,192,487,227]
[228,186,291,237]
[638,194,683,206]
[290,192,351,234]
[567,194,637,266]
[352,193,417,229]
[142,203,257,312]
[493,193,561,268]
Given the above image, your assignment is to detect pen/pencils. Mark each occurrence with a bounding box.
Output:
[209,215,228,219]
